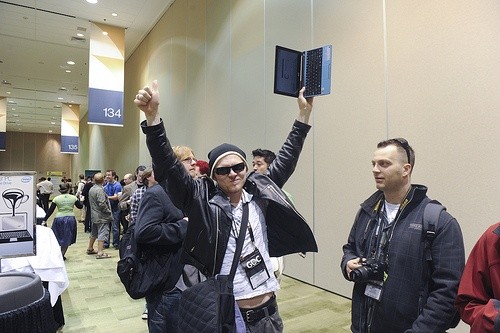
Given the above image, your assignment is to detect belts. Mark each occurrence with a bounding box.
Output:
[240,295,276,323]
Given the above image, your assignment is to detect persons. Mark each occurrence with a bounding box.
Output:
[250,148,284,285]
[456,221,500,333]
[86,172,114,259]
[134,79,318,333]
[128,167,155,319]
[103,166,147,249]
[195,160,210,178]
[60,174,95,233]
[341,138,465,333]
[41,182,85,261]
[134,146,195,331]
[36,177,54,213]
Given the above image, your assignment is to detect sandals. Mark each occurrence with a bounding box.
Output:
[96,252,111,259]
[86,249,98,254]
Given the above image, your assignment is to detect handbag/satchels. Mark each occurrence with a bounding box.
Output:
[176,274,235,333]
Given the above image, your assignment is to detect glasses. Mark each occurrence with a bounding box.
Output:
[183,157,198,164]
[214,162,245,175]
[393,137,411,164]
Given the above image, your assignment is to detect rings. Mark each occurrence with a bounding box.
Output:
[136,94,140,99]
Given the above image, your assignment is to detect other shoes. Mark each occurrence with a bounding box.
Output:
[103,245,106,250]
[141,309,148,319]
[114,245,119,250]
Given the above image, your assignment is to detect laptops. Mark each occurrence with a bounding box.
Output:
[274,45,332,98]
[0,212,33,243]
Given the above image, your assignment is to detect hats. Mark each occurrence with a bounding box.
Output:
[207,143,249,179]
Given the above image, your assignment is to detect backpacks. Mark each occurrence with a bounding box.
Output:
[116,219,183,300]
[422,200,461,329]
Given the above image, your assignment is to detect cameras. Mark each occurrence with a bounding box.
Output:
[349,259,384,284]
